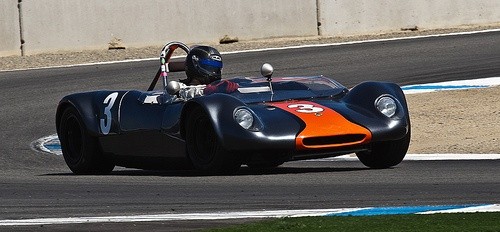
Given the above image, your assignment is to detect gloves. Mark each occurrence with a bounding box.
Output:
[204,80,238,94]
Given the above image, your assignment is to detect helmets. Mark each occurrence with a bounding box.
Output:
[185,46,223,82]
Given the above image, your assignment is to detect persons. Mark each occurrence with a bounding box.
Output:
[166,46,238,101]
